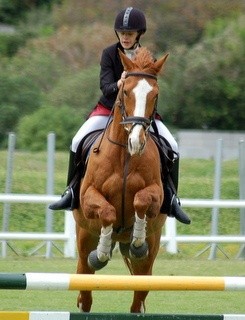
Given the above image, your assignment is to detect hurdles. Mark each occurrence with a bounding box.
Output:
[0,272,245,320]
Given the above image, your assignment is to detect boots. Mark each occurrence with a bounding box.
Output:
[48,148,80,210]
[161,157,191,224]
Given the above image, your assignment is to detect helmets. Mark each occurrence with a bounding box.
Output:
[113,7,147,31]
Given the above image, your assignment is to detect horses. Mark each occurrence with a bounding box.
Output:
[73,47,180,313]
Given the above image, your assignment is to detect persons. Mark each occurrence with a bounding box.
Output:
[48,7,192,224]
